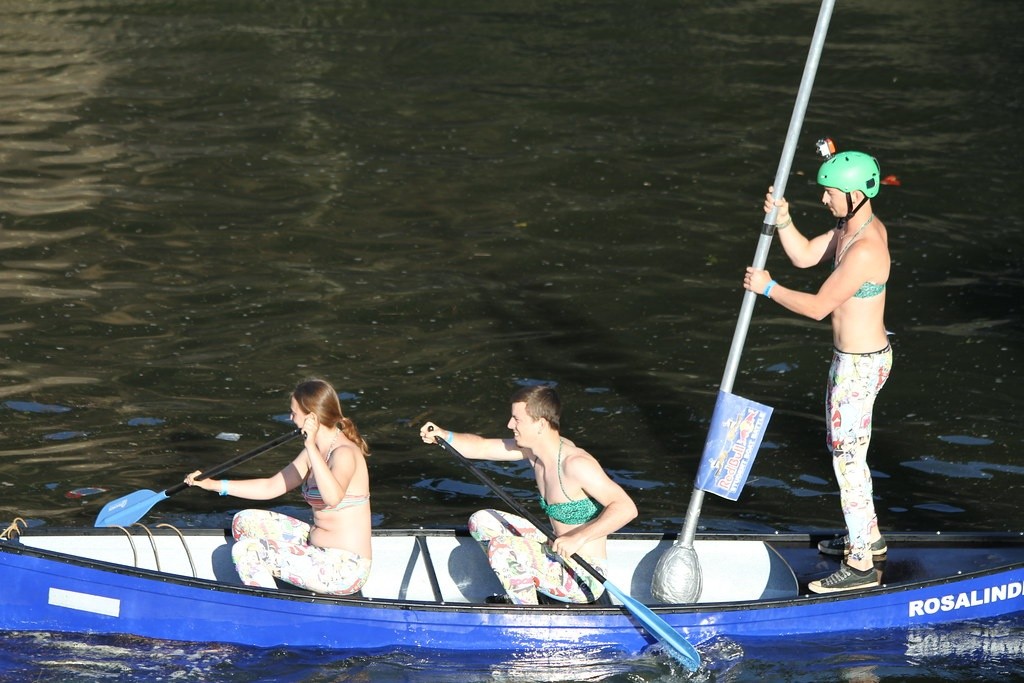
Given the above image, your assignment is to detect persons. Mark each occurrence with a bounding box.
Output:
[184,378,371,595]
[742,150,892,593]
[419,384,638,604]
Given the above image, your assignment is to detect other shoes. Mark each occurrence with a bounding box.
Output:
[482,593,512,603]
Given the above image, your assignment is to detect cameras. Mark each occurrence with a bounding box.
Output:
[816,138,837,156]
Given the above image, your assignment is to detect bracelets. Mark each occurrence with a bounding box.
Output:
[219,480,228,496]
[776,217,792,229]
[764,280,776,297]
[447,431,454,444]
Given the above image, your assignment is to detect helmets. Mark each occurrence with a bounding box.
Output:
[816,151,880,198]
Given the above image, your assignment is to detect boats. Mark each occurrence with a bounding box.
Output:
[0,528,1024,654]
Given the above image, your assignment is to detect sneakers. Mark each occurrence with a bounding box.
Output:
[817,533,888,555]
[809,560,879,593]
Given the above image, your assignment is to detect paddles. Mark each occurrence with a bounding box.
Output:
[427,423,701,674]
[94,426,304,528]
